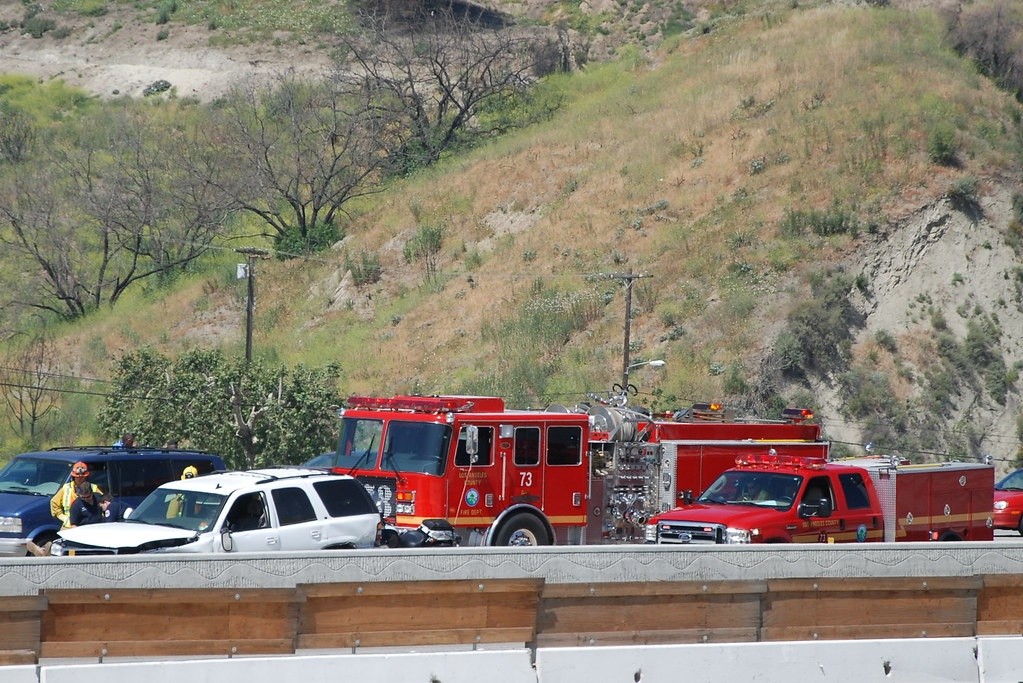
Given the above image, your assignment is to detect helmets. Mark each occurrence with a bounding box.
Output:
[70,462,89,476]
[180,465,197,480]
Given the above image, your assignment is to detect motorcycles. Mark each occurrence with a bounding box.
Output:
[380,516,462,551]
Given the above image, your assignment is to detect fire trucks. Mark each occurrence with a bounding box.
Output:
[328,390,834,552]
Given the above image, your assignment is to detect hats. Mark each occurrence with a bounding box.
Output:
[166,439,178,445]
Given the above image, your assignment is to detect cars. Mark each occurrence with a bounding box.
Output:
[990,468,1023,536]
[302,449,389,475]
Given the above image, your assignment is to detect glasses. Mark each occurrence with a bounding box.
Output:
[81,495,90,498]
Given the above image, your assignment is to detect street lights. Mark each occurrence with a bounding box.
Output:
[621,360,666,405]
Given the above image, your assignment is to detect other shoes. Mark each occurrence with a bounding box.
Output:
[26,540,47,556]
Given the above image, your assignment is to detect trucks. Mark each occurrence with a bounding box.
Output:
[642,453,996,545]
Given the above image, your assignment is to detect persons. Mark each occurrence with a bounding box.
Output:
[165,465,201,519]
[27,538,64,556]
[50,462,104,531]
[97,494,131,523]
[113,434,137,447]
[69,483,105,527]
[736,479,769,502]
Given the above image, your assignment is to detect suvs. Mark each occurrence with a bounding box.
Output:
[48,463,384,559]
[0,446,229,558]
